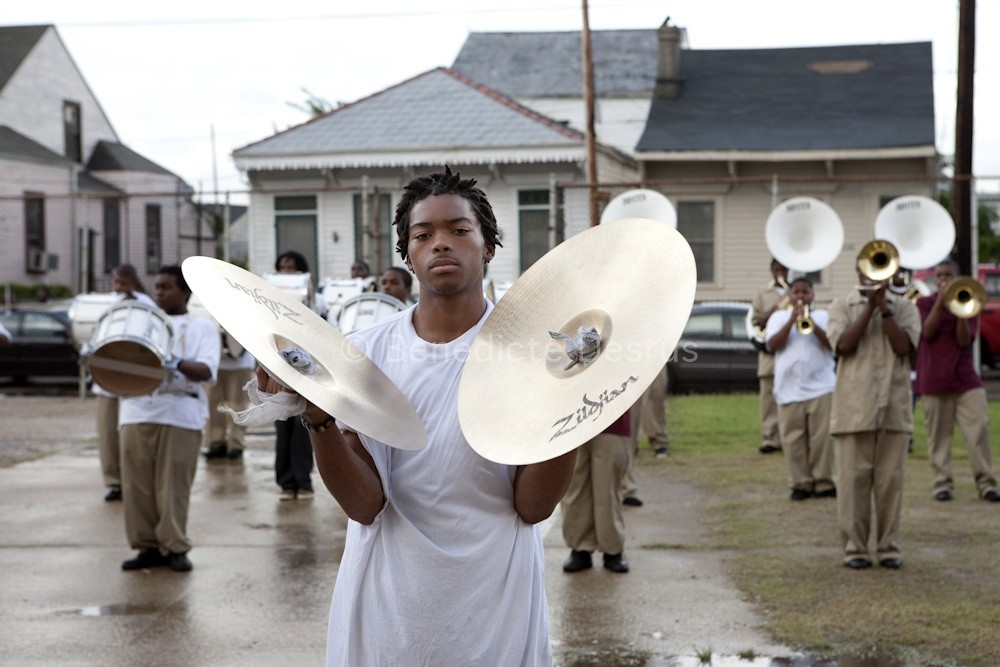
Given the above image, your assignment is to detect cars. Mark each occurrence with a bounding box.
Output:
[0,290,123,395]
[912,263,1000,371]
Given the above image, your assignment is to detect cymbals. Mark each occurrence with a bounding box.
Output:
[180,255,429,451]
[454,217,698,466]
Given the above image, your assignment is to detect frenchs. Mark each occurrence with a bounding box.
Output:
[745,195,846,354]
[873,194,956,306]
[601,189,678,229]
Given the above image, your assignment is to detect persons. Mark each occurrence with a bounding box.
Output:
[273,251,318,499]
[884,259,1000,503]
[256,164,580,667]
[87,264,254,571]
[630,362,670,462]
[826,254,921,569]
[559,405,631,573]
[352,259,413,305]
[746,255,793,453]
[766,277,837,498]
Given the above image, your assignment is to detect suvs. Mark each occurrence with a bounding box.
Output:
[669,299,765,394]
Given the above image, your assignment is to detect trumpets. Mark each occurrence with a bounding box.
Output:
[855,239,902,293]
[792,300,815,336]
[942,277,988,320]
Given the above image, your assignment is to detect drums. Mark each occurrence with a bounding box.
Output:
[321,276,408,334]
[187,295,246,360]
[67,291,175,399]
[261,271,315,309]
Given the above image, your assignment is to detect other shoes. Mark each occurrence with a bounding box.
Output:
[881,557,904,569]
[279,489,294,500]
[936,491,952,501]
[297,490,314,500]
[623,497,642,506]
[160,551,193,572]
[204,445,228,460]
[122,552,160,570]
[790,488,812,500]
[813,480,835,497]
[984,490,999,503]
[563,550,592,572]
[848,558,872,569]
[104,491,121,502]
[228,449,242,460]
[656,449,668,460]
[760,446,779,454]
[603,553,628,572]
